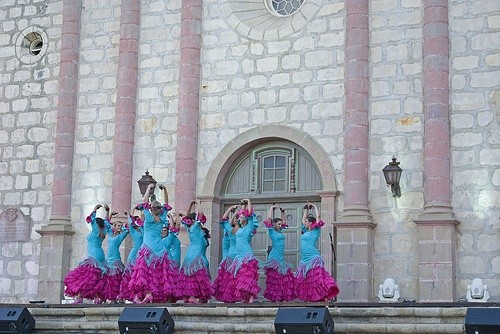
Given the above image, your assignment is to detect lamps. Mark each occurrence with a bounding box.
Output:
[467,278,491,302]
[377,278,403,303]
[137,168,156,202]
[382,155,402,212]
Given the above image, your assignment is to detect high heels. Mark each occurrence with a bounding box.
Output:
[140,294,154,303]
[188,296,202,304]
[93,298,99,304]
[68,297,83,305]
[133,296,142,303]
[99,298,107,304]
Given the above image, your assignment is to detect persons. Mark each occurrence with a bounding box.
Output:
[260,206,297,302]
[175,200,214,303]
[294,202,339,302]
[62,203,111,304]
[116,204,147,303]
[201,227,211,271]
[128,184,176,305]
[212,199,259,303]
[106,211,130,304]
[161,213,181,279]
[170,211,181,266]
